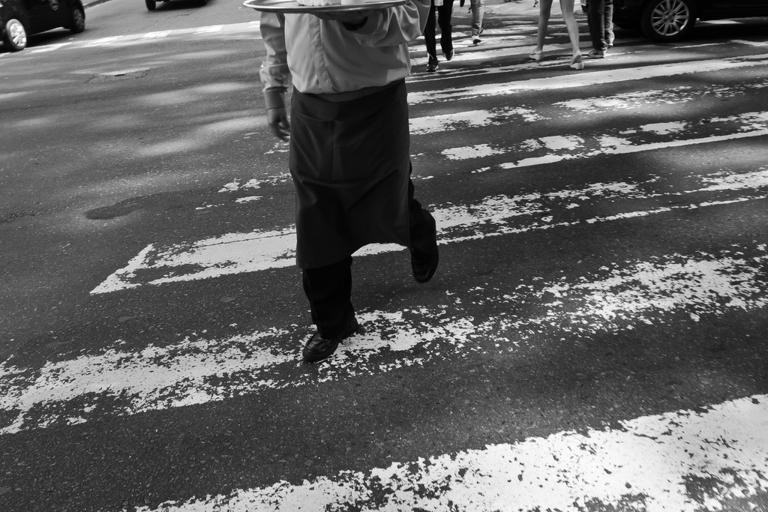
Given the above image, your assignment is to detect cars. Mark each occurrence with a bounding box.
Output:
[0,0,85,50]
[581,1,768,43]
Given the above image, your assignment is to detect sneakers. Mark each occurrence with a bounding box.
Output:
[588,34,614,58]
[303,316,358,362]
[472,35,481,44]
[426,40,454,72]
[411,213,438,283]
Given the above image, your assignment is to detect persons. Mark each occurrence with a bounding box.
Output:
[260,0,439,362]
[529,0,584,69]
[471,0,485,44]
[424,0,454,71]
[585,0,615,58]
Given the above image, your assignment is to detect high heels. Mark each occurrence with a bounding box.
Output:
[529,50,544,60]
[570,62,585,69]
